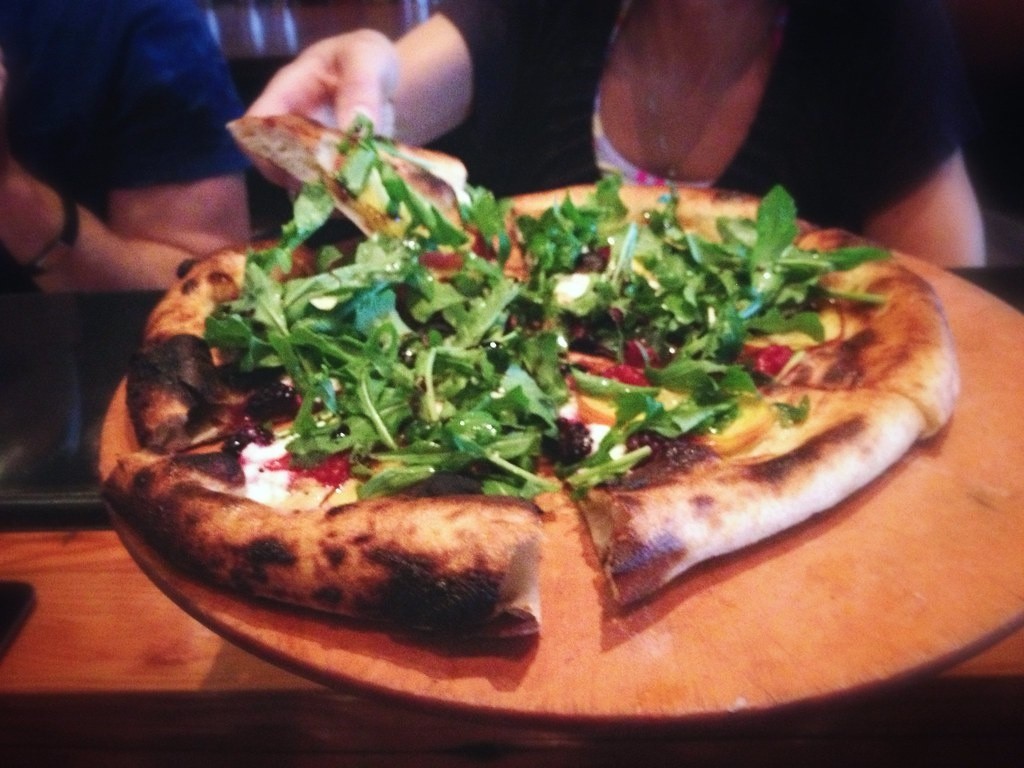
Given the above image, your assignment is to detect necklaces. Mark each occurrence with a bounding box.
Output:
[621,24,767,179]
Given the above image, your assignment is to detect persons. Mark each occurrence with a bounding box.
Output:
[0,0,256,294]
[244,0,988,269]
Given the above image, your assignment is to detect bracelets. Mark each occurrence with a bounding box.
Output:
[26,196,79,293]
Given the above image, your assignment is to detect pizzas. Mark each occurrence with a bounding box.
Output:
[100,113,960,636]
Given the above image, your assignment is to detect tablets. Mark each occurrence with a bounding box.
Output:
[1,290,169,534]
